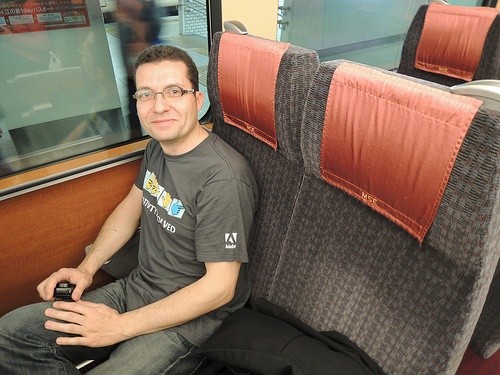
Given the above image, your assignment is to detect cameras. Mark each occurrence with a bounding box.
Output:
[54,280,75,300]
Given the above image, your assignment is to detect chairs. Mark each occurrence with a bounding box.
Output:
[85,21,500,375]
[396,0,500,87]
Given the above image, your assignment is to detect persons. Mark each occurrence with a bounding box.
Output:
[116,0,162,142]
[0,46,257,375]
[64,23,139,143]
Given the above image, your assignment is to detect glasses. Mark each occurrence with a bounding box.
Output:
[76,44,97,65]
[131,85,194,103]
